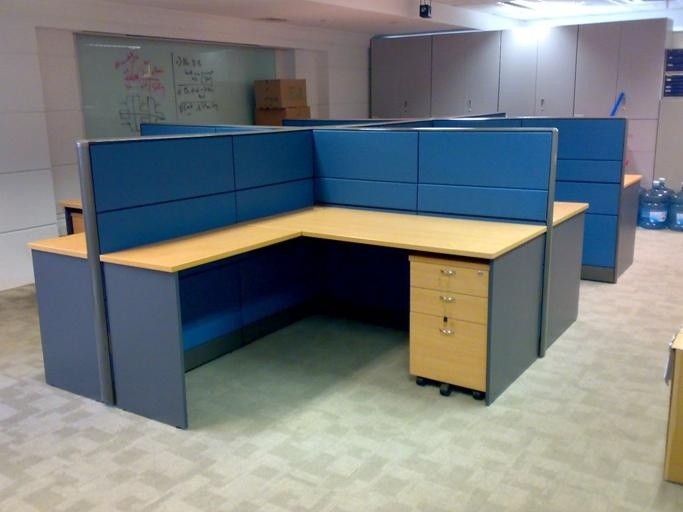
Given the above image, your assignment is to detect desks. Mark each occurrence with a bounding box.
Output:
[545,199,589,355]
[29,193,97,402]
[616,173,641,279]
[99,198,547,428]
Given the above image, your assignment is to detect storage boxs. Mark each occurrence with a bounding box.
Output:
[254,108,310,128]
[251,79,308,108]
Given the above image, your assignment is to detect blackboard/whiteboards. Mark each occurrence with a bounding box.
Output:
[74,29,276,141]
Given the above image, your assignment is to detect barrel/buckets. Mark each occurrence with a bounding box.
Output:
[639,178,682,229]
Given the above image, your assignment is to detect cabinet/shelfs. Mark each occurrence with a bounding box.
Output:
[431,29,502,120]
[660,326,682,488]
[499,24,581,121]
[371,35,433,123]
[576,15,673,192]
